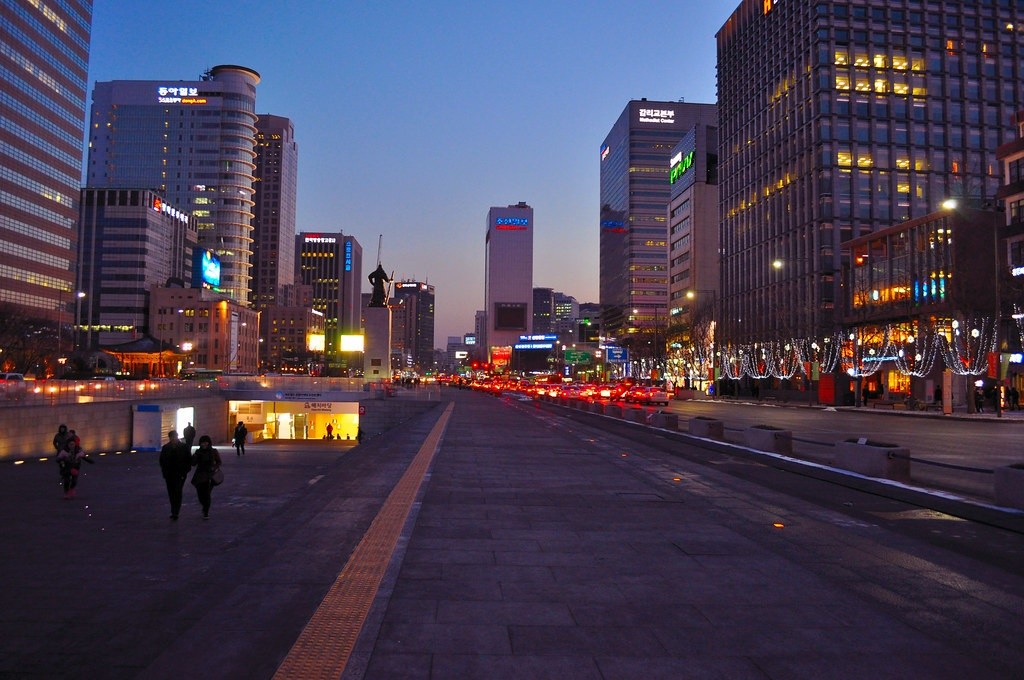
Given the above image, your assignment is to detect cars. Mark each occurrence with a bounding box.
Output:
[394,371,669,406]
[139,371,295,401]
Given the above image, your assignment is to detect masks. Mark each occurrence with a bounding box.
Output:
[200,446,206,449]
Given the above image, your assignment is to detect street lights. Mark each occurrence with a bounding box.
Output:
[58,290,86,379]
[771,256,862,408]
[938,197,1001,419]
[633,306,659,387]
[686,288,720,400]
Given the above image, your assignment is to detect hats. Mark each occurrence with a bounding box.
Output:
[237,422,243,425]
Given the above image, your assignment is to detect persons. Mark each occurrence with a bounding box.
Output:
[751,384,759,399]
[53,425,94,499]
[327,423,333,440]
[691,386,697,390]
[402,378,421,388]
[862,385,870,406]
[975,386,1020,413]
[235,422,247,456]
[935,385,942,411]
[358,427,363,444]
[459,378,462,390]
[368,265,393,307]
[323,434,350,440]
[159,431,191,520]
[191,435,221,519]
[184,422,195,447]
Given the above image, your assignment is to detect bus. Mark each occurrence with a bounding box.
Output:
[176,367,223,383]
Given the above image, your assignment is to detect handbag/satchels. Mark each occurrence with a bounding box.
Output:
[212,467,224,486]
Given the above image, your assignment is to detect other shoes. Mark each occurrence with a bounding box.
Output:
[169,514,179,522]
[70,488,76,496]
[64,491,70,498]
[200,509,210,519]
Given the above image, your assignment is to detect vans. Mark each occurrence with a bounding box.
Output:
[0,372,27,400]
[88,376,118,395]
